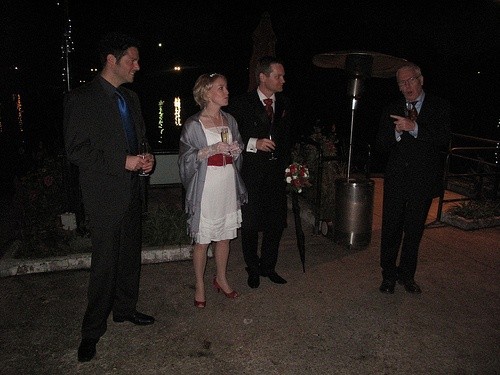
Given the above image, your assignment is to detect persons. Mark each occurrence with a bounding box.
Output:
[231,57,294,288]
[63,36,156,362]
[376,63,453,293]
[178,71,250,310]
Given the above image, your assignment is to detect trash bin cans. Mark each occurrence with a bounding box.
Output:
[334,177,375,249]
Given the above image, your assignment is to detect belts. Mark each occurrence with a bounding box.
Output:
[207,153,233,166]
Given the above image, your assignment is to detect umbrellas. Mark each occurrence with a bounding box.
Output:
[291,190,306,273]
[246,12,277,97]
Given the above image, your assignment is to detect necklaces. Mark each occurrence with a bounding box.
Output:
[202,108,223,134]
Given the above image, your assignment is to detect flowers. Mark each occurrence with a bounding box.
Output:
[285,162,312,191]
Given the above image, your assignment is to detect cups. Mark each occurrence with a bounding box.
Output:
[403,102,411,119]
[221,128,229,145]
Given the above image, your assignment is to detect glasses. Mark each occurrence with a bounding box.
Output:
[397,76,420,87]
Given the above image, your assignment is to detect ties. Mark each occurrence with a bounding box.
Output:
[113,89,136,147]
[263,98,275,124]
[407,101,418,122]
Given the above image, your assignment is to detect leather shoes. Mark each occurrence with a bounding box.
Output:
[113,309,155,325]
[78,337,99,362]
[381,279,396,293]
[397,278,421,294]
[247,274,260,288]
[260,270,287,284]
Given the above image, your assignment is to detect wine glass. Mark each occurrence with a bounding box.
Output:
[137,142,149,177]
[266,134,277,160]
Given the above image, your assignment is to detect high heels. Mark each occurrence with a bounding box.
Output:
[193,298,206,309]
[213,278,239,299]
